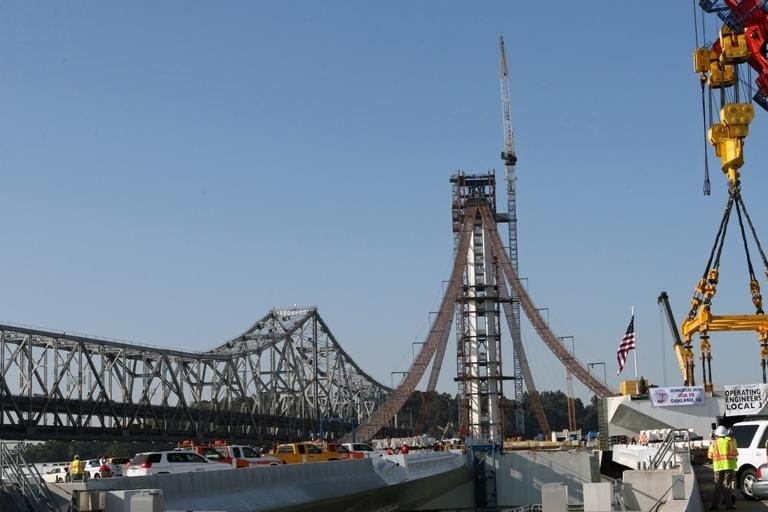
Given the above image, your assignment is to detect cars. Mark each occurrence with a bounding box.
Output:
[749,461,768,500]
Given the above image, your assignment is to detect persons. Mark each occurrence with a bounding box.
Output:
[706,424,738,510]
[629,434,638,445]
[400,440,410,453]
[639,431,650,445]
[720,429,738,509]
[67,454,84,482]
[99,458,110,478]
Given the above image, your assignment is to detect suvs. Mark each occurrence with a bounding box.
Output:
[725,419,768,502]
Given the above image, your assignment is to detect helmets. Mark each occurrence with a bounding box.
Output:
[715,426,728,437]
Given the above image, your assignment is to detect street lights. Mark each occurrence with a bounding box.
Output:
[406,407,413,429]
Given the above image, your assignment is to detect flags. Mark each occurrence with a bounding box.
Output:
[616,315,636,378]
